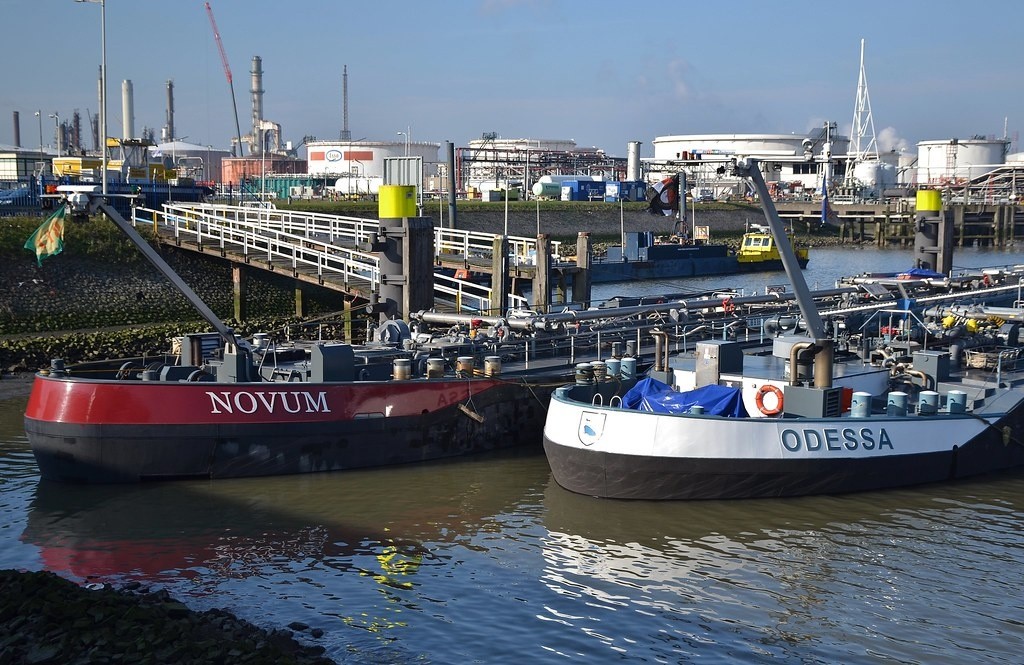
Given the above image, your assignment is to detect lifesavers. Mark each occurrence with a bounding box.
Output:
[756,385,784,416]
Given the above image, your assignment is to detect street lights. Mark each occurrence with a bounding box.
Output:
[348,137,367,201]
[48,113,61,158]
[397,131,407,157]
[536,199,544,236]
[207,144,213,183]
[171,137,183,164]
[34,109,44,173]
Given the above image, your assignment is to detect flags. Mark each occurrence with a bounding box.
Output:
[24,203,65,268]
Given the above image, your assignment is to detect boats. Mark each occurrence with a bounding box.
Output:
[18,146,1023,502]
[727,216,811,273]
